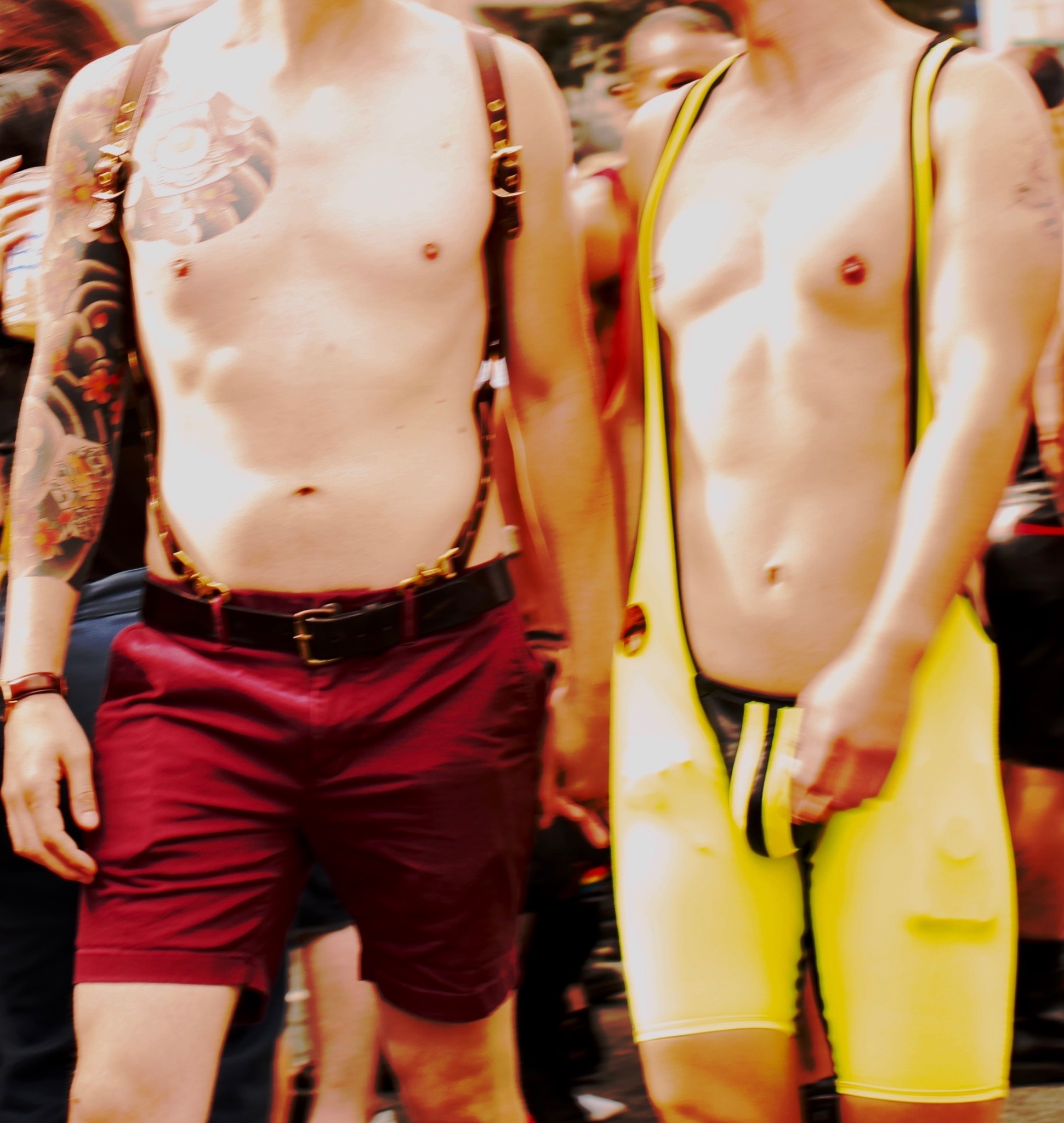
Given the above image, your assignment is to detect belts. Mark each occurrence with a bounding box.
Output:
[139,557,516,664]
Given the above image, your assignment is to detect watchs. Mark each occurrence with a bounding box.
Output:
[0,671,70,725]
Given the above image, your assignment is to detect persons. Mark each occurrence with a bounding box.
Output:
[0,0,1063,1123]
